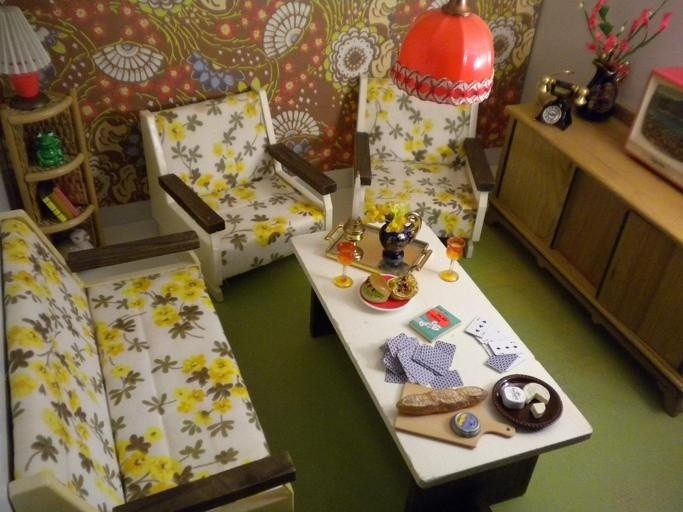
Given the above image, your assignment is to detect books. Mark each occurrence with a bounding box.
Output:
[39,193,68,222]
[45,188,72,220]
[51,182,80,217]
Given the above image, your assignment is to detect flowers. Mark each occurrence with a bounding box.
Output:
[578,1,676,71]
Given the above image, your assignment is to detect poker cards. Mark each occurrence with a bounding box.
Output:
[466,316,518,371]
[381,333,464,389]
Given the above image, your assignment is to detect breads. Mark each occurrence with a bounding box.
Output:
[395,385,489,414]
[502,379,551,418]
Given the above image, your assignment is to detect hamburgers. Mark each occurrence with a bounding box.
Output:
[361,274,391,303]
[387,275,418,300]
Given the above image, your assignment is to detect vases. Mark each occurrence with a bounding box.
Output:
[573,56,619,122]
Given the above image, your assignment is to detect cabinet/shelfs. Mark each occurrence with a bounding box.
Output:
[0,88,106,260]
[484,95,683,417]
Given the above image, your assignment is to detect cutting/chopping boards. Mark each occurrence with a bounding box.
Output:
[394,382,517,449]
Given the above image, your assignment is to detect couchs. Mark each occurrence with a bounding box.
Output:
[0,209,296,512]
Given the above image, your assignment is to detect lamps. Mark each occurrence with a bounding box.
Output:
[0,5,52,111]
[389,1,494,107]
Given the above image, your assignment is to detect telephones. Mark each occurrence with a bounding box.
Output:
[535,76,590,131]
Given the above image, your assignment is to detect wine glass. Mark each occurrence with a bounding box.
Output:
[331,240,356,289]
[438,235,466,283]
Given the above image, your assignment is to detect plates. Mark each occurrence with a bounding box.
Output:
[358,274,410,311]
[492,373,563,431]
